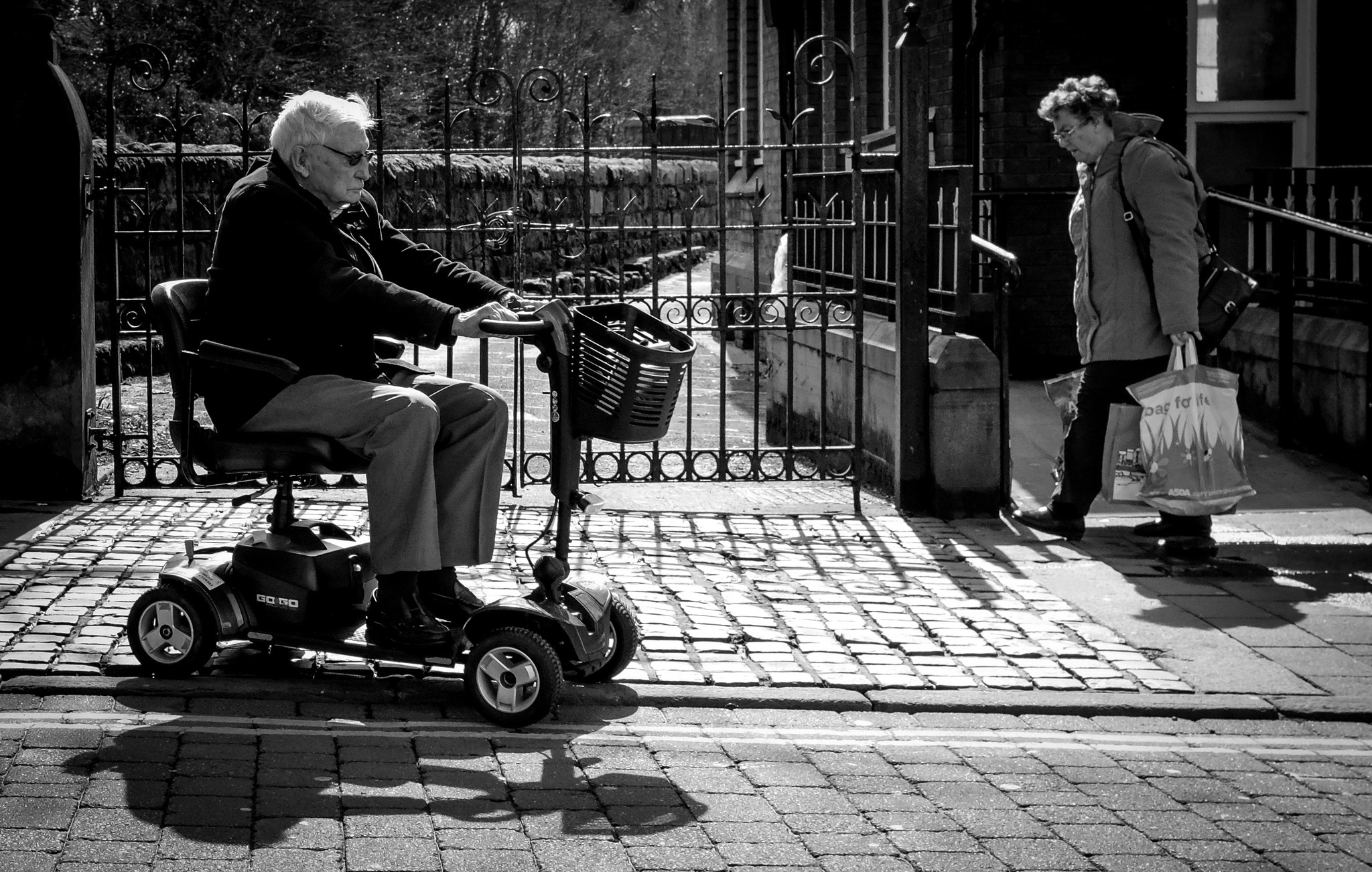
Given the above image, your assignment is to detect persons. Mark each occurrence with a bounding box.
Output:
[1011,76,1217,551]
[202,89,533,655]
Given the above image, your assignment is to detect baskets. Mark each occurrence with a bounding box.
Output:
[568,303,696,445]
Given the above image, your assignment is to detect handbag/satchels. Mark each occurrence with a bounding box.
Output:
[1155,253,1258,360]
[1099,402,1239,518]
[1127,334,1258,516]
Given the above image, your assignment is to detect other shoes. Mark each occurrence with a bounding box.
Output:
[1012,506,1086,541]
[1132,517,1213,544]
[365,588,460,651]
[416,580,485,625]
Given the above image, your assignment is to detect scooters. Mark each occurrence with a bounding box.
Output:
[127,277,702,729]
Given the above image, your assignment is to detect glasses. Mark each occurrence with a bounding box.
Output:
[1049,117,1089,142]
[321,145,375,166]
